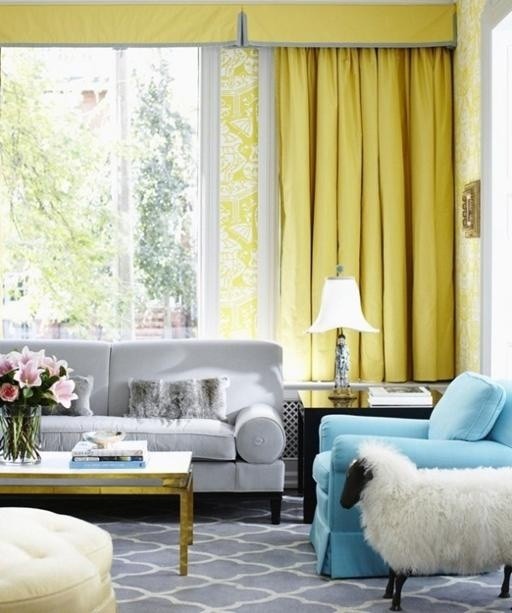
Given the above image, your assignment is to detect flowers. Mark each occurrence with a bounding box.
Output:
[0,344,76,457]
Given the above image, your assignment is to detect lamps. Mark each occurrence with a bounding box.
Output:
[305,264,383,401]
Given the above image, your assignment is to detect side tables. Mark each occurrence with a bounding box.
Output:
[297,383,433,521]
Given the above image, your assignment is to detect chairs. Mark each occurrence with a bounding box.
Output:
[310,367,512,582]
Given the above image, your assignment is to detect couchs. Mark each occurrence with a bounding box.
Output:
[0,337,286,525]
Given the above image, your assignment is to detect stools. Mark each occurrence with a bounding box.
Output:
[0,504,117,613]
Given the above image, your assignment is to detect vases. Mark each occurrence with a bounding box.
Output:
[2,405,43,468]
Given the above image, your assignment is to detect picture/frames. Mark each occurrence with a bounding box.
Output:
[461,180,479,239]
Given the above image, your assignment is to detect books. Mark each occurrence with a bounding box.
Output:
[368,386,433,405]
[69,440,151,468]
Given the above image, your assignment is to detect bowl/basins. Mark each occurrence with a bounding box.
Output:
[82,431,125,448]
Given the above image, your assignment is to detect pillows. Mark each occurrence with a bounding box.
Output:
[426,372,507,443]
[52,374,95,417]
[126,371,229,422]
